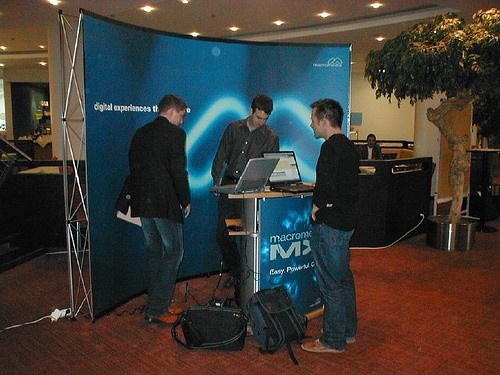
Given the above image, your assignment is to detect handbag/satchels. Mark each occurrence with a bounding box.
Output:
[172,306,248,353]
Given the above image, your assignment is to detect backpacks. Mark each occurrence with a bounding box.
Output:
[245,286,309,355]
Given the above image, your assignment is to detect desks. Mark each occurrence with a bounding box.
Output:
[466,149,500,233]
[225,182,324,335]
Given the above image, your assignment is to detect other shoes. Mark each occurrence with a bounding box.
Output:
[143,316,171,328]
[301,339,346,354]
[320,327,356,344]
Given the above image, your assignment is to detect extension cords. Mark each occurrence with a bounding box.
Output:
[51,308,72,319]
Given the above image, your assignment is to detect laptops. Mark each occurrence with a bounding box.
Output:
[262,151,315,193]
[209,157,280,194]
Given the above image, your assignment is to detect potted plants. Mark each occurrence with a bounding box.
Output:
[365,7,500,252]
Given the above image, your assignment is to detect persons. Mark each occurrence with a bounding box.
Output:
[128,95,192,325]
[301,98,359,352]
[211,93,279,289]
[358,134,383,160]
